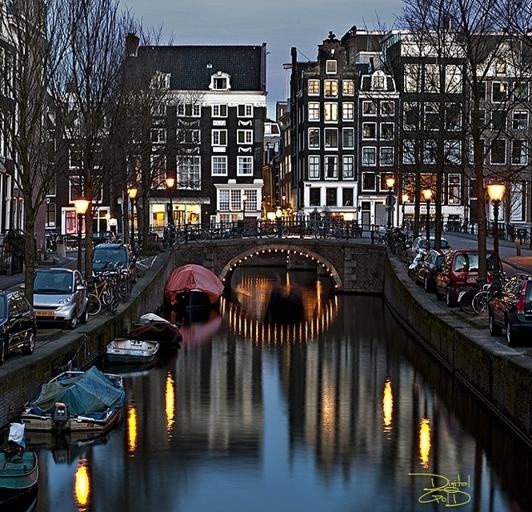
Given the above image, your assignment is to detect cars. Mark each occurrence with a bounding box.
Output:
[33,268,89,329]
[92,244,132,276]
[64,230,164,247]
[0,290,37,363]
[489,273,532,346]
[407,236,503,305]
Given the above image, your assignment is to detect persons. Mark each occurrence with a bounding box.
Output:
[310,206,321,234]
[296,205,305,240]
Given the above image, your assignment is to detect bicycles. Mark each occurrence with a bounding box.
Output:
[89,260,131,316]
[177,216,343,244]
[383,227,399,254]
[458,271,509,316]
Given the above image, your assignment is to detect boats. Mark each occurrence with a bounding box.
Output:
[0,451,38,504]
[176,289,210,309]
[20,371,123,444]
[106,313,179,364]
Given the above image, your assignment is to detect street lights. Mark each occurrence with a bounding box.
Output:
[402,195,408,226]
[242,196,247,219]
[74,196,89,273]
[421,186,432,250]
[386,179,395,227]
[166,178,175,246]
[488,180,505,274]
[127,187,138,249]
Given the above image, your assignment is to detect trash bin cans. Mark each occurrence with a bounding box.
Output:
[56,240,66,258]
[517,229,528,239]
